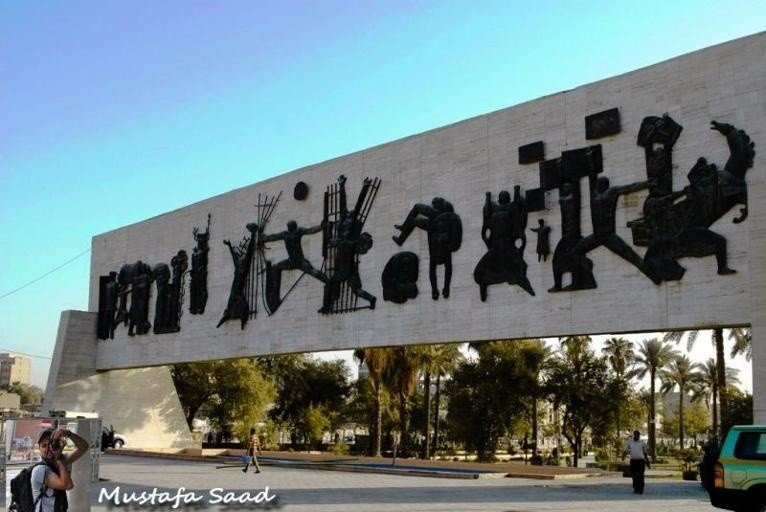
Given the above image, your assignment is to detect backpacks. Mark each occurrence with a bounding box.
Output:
[10,462,49,512]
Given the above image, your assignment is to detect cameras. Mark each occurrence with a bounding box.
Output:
[50,440,60,451]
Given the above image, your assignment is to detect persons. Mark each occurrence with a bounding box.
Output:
[242,428,262,473]
[622,430,651,496]
[10,428,89,512]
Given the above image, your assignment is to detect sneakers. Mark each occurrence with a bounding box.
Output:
[242,468,248,472]
[254,469,260,473]
[242,456,251,463]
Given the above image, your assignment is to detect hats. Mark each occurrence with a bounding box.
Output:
[38,427,54,443]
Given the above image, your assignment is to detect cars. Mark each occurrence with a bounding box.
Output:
[101,426,126,452]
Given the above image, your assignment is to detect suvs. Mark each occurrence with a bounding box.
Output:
[699,426,766,511]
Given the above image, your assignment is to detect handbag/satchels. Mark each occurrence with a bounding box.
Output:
[622,465,632,478]
